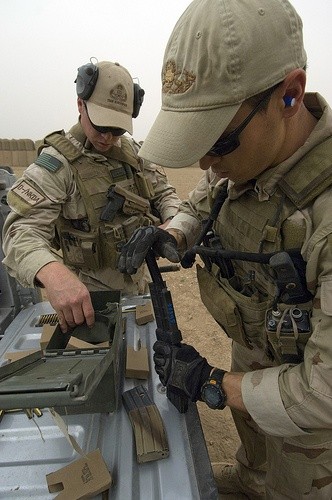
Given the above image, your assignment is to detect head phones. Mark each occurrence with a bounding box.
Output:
[73,56,145,118]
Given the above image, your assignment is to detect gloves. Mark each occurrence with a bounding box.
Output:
[118,227,177,275]
[154,341,207,401]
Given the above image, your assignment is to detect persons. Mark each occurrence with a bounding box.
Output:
[1,60,183,334]
[137,0,332,500]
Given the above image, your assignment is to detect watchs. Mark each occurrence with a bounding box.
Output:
[200,366,227,410]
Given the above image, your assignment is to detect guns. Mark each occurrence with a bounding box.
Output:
[101,183,152,223]
[143,248,189,413]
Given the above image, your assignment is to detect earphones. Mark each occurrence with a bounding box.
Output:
[284,95,295,106]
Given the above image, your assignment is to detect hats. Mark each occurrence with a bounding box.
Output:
[137,0,307,168]
[84,61,134,136]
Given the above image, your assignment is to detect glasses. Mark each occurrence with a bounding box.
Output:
[84,103,126,137]
[205,93,271,157]
